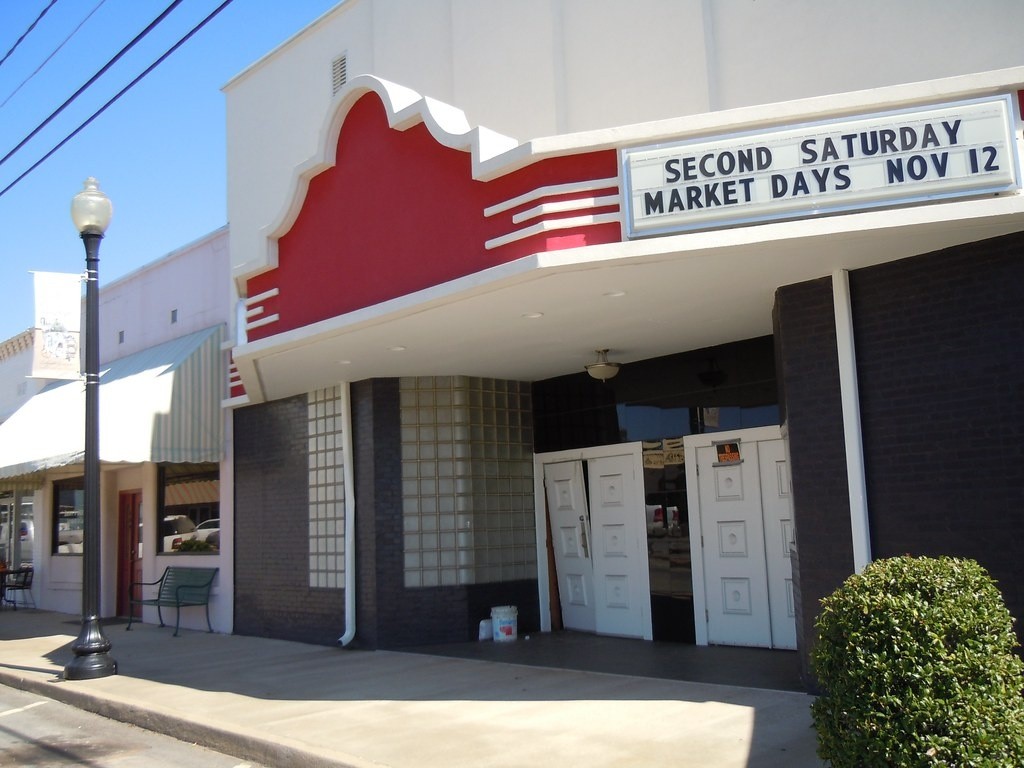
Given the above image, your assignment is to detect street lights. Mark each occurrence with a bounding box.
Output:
[60,175,120,683]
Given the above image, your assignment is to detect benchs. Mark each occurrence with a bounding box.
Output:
[127,566,219,638]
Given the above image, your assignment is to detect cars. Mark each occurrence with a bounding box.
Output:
[0,501,225,558]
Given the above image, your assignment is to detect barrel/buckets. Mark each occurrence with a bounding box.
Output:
[490,605,518,643]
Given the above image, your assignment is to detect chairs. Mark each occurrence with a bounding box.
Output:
[8,565,35,609]
[6,563,28,608]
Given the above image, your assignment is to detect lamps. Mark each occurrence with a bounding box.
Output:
[585,350,620,383]
